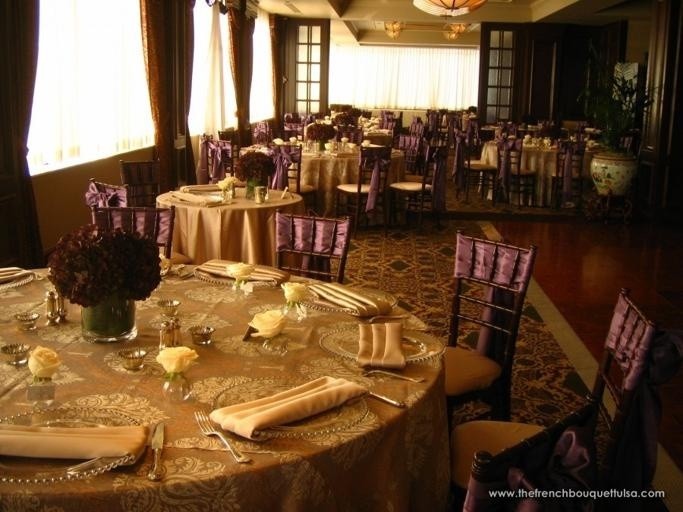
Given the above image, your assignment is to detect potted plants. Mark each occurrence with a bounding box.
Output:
[575,60,665,196]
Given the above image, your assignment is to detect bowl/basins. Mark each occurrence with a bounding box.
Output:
[188,325,216,344]
[157,298,182,315]
[119,348,148,370]
[0,343,31,365]
[14,312,40,331]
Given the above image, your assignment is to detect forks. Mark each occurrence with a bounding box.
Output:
[352,312,408,324]
[193,410,251,463]
[341,356,425,384]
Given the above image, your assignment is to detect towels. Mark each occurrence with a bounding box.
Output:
[0,422,149,462]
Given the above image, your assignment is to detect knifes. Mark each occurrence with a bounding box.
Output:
[146,421,165,481]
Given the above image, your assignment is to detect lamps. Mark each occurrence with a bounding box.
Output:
[413,0,488,18]
[385,21,466,41]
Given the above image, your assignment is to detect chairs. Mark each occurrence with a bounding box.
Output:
[462,395,601,512]
[449,287,683,512]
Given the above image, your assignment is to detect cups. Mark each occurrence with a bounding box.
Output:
[254,187,267,203]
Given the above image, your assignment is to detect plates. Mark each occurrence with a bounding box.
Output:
[319,326,446,364]
[167,193,224,205]
[299,287,398,313]
[0,273,34,290]
[211,378,369,438]
[0,405,141,483]
[194,270,277,288]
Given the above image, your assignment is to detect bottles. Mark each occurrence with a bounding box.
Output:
[169,317,183,347]
[56,292,68,324]
[160,320,171,350]
[45,290,60,327]
